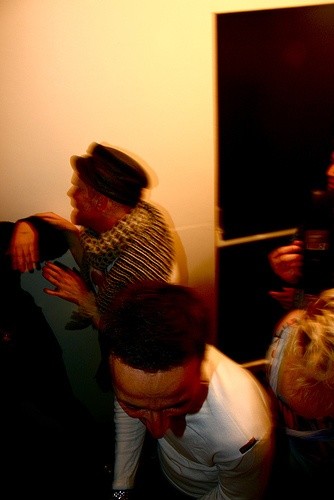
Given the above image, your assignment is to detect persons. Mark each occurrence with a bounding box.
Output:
[97,281,278,500]
[268,147,334,310]
[263,289,334,500]
[11,141,192,330]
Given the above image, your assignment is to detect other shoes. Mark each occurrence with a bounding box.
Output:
[104,463,144,472]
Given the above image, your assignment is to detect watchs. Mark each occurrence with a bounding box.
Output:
[110,489,130,500]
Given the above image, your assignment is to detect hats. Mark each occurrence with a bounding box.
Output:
[70,142,148,208]
